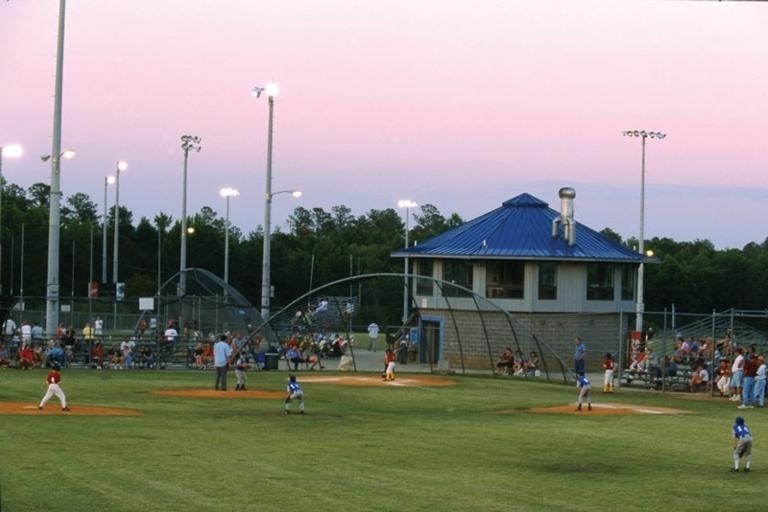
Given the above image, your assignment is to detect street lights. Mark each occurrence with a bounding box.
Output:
[177,134,203,301]
[619,125,665,331]
[251,85,303,321]
[100,159,130,288]
[218,186,240,303]
[0,143,22,176]
[632,244,654,333]
[41,145,77,344]
[397,196,416,328]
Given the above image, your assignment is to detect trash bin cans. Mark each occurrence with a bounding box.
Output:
[264,353,279,371]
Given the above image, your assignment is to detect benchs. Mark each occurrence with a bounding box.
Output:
[0,332,350,371]
[618,338,736,393]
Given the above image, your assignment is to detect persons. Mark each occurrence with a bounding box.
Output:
[341,298,355,317]
[367,321,379,352]
[495,347,513,374]
[602,354,613,393]
[382,350,397,381]
[234,351,250,391]
[37,365,71,411]
[384,349,392,375]
[311,298,329,316]
[730,416,752,473]
[524,352,538,374]
[574,338,586,379]
[575,369,591,411]
[606,357,617,387]
[512,350,522,374]
[282,373,305,415]
[384,324,396,350]
[629,325,767,410]
[212,335,232,390]
